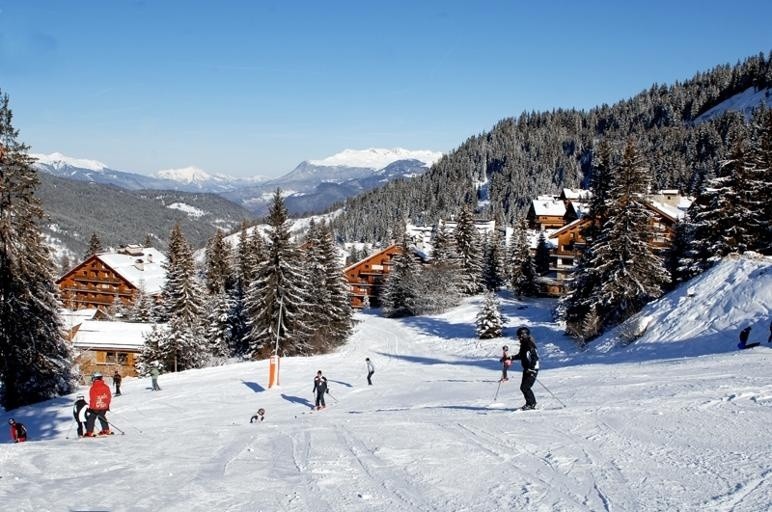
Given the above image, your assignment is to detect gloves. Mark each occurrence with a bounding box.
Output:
[500,356,511,363]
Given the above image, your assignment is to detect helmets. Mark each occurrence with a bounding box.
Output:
[91,372,103,380]
[517,327,530,340]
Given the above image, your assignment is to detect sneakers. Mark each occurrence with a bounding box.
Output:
[523,403,536,410]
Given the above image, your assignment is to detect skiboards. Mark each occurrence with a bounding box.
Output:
[476,407,562,415]
[302,402,333,415]
[79,431,114,437]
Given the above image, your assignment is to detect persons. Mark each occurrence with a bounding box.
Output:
[8,418,29,442]
[86,373,113,437]
[498,327,541,411]
[113,370,122,397]
[251,408,264,423]
[501,346,511,379]
[148,363,160,390]
[739,327,752,346]
[73,394,91,438]
[365,357,375,387]
[312,369,331,409]
